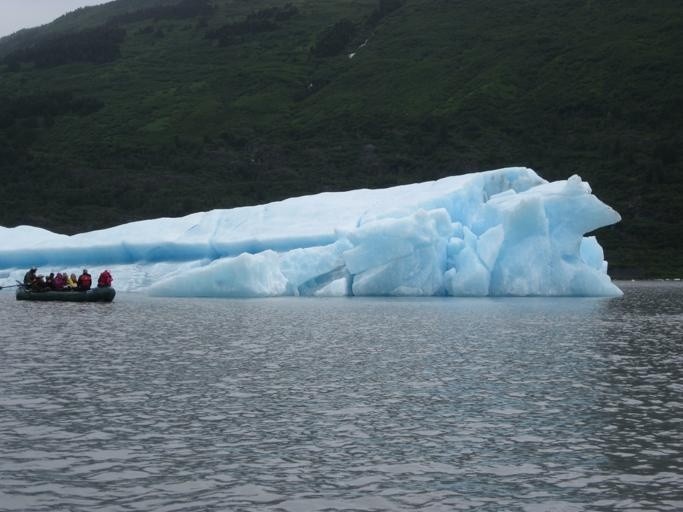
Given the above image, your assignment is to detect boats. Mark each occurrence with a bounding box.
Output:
[14,286,116,303]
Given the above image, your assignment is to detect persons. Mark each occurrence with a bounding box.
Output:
[20,266,113,294]
